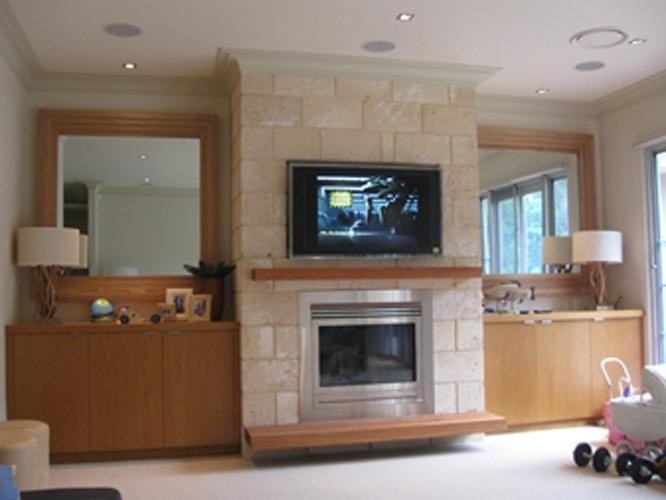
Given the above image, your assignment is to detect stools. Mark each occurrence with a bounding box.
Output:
[0,420,51,491]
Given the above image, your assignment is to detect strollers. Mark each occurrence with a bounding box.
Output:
[597,351,666,457]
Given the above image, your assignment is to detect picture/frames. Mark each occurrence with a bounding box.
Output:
[185,294,212,322]
[165,287,192,319]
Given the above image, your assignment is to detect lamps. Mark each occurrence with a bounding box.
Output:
[571,230,622,309]
[17,226,80,323]
[544,236,581,273]
[58,236,88,276]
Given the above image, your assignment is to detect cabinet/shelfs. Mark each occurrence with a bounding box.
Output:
[484,308,647,429]
[6,320,240,465]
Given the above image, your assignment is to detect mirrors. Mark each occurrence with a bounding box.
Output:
[475,128,596,294]
[36,107,221,302]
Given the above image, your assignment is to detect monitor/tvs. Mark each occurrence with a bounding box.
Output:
[293,168,438,254]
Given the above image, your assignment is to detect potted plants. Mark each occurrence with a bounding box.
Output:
[183,259,236,321]
